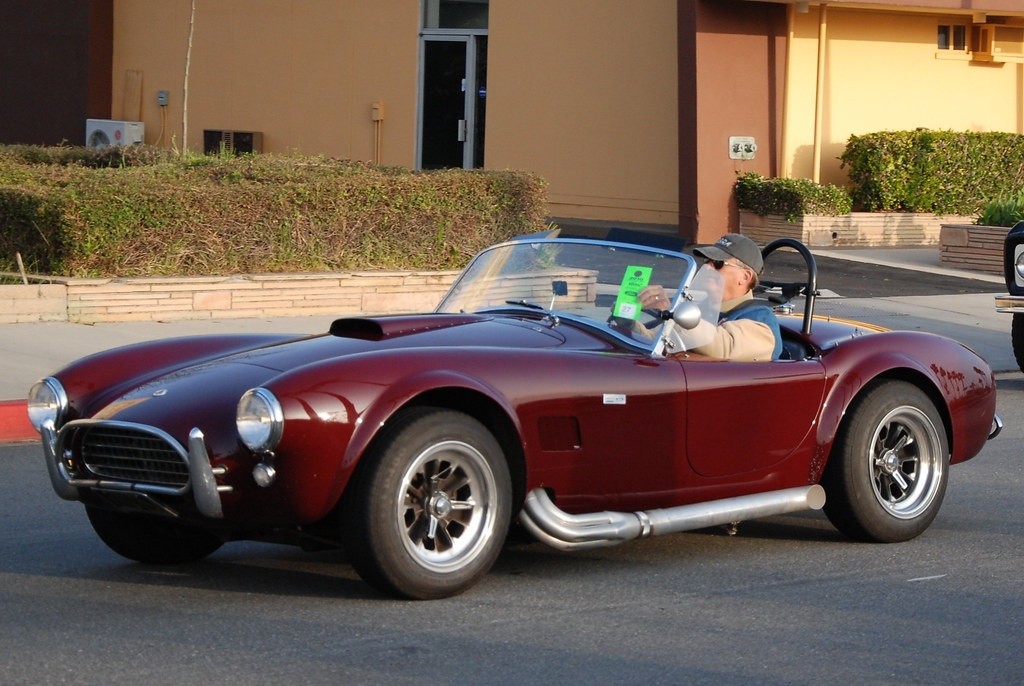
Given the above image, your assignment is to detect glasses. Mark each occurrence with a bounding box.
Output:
[702,256,740,271]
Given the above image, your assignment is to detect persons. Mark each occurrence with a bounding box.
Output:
[611,235,783,362]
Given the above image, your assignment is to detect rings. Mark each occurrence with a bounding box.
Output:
[654,295,660,303]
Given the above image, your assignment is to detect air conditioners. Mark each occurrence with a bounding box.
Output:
[86,118,145,148]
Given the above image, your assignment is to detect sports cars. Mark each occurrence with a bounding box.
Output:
[27,240,1003,596]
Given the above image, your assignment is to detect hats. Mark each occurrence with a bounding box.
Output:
[693,232,767,275]
